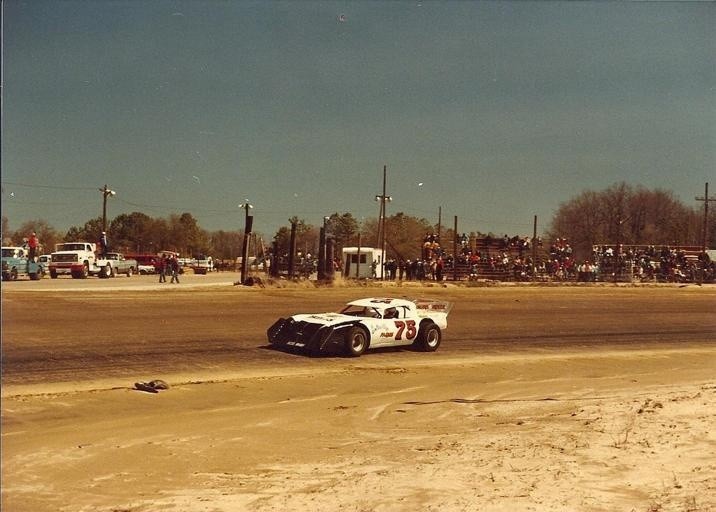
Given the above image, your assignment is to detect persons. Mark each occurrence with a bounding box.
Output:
[99,232,108,259]
[27,232,38,264]
[95,242,101,260]
[196,250,343,278]
[374,306,398,319]
[372,260,378,280]
[169,253,180,284]
[157,252,169,282]
[385,231,716,286]
[18,237,28,249]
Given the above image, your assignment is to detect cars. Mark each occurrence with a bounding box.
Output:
[138,265,156,275]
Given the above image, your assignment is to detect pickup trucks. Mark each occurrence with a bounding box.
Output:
[106,251,138,277]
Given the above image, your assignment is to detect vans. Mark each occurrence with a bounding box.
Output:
[37,255,51,271]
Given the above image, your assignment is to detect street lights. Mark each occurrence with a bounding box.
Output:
[102,185,117,231]
[374,196,392,277]
[238,199,253,281]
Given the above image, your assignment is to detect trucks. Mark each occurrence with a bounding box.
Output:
[48,242,116,279]
[0,246,44,281]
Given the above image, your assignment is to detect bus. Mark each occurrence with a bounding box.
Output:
[124,255,164,274]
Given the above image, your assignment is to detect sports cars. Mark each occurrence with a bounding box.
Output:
[268,297,455,357]
